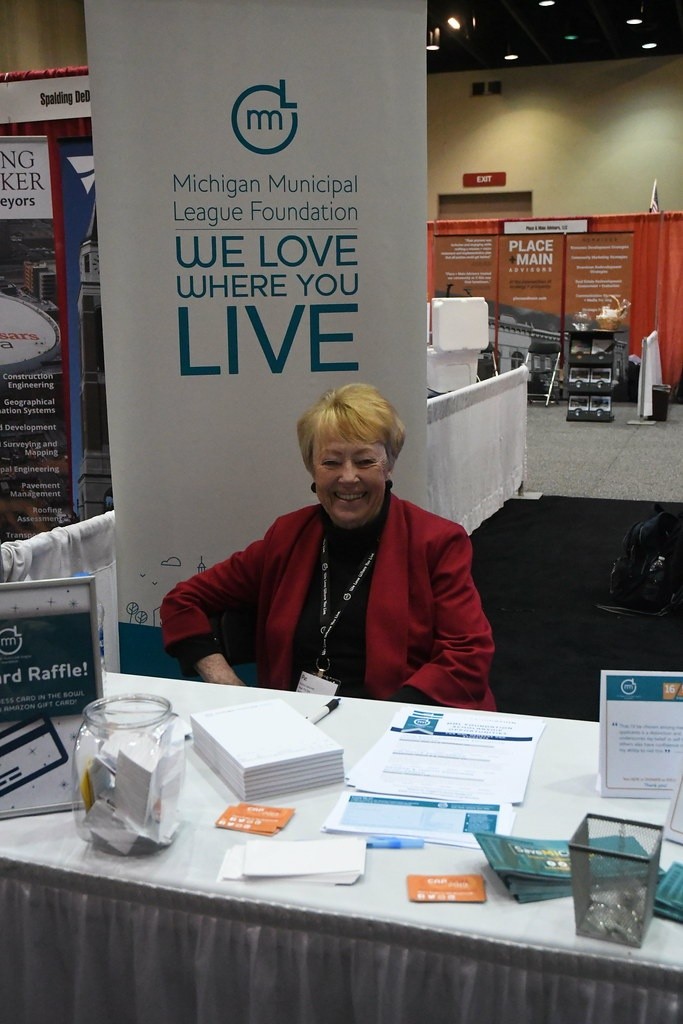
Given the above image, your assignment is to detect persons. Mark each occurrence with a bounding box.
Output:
[157,380,498,710]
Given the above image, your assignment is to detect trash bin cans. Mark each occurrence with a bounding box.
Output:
[651,383,672,421]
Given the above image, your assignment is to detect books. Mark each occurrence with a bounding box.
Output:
[185,693,347,800]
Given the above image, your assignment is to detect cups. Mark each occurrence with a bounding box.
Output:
[71,691,193,858]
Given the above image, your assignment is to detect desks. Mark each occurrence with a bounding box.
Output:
[1,670,682,1023]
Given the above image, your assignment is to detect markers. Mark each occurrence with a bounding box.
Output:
[306,697,341,724]
